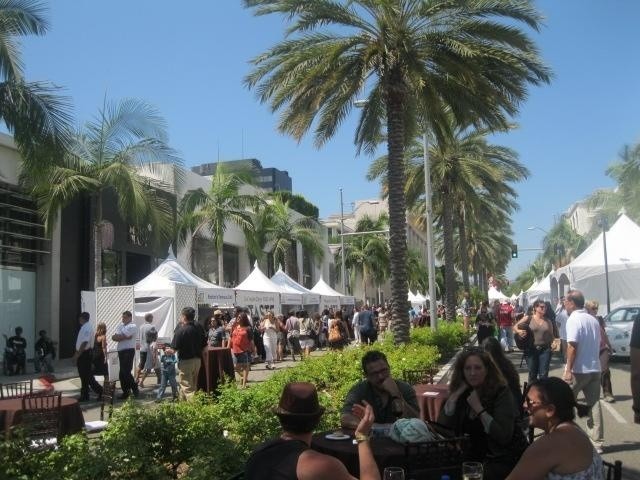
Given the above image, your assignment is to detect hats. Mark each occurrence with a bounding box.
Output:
[271,381,325,417]
[213,310,223,315]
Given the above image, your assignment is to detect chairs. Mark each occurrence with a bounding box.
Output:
[0,382,18,399]
[403,432,471,478]
[602,459,623,479]
[521,381,537,445]
[17,390,63,438]
[16,378,34,397]
[96,378,117,420]
[401,368,434,386]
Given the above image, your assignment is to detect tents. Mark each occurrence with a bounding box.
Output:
[517,212,640,300]
[132,244,354,308]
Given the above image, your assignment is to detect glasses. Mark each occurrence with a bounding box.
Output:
[525,396,545,408]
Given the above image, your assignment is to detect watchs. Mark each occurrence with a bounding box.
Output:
[357,434,370,442]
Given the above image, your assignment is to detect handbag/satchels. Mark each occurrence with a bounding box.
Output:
[289,319,299,338]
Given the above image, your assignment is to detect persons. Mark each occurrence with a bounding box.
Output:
[244,383,381,480]
[480,337,523,419]
[242,307,253,327]
[76,313,103,403]
[461,293,471,332]
[505,377,607,480]
[438,346,529,453]
[319,309,330,348]
[134,313,162,384]
[113,312,141,400]
[328,310,351,351]
[475,307,495,343]
[276,314,285,361]
[226,311,255,388]
[327,311,334,349]
[341,351,420,432]
[476,300,493,316]
[512,300,524,346]
[172,307,208,348]
[299,310,315,360]
[156,344,179,403]
[378,307,388,335]
[556,296,566,316]
[263,311,276,369]
[411,305,447,328]
[168,309,202,403]
[34,330,55,373]
[586,300,615,403]
[207,316,226,347]
[285,310,304,362]
[312,312,321,348]
[497,301,515,353]
[630,310,640,424]
[564,291,605,454]
[351,308,361,346]
[342,307,348,321]
[139,327,161,387]
[260,311,279,370]
[359,304,377,346]
[7,326,27,374]
[92,323,109,381]
[543,301,557,337]
[555,302,569,364]
[224,306,251,371]
[512,301,555,387]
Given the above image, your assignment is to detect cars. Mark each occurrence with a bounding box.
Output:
[603,305,640,360]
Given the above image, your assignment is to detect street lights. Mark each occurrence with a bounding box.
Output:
[526,225,558,263]
[554,244,564,268]
[596,216,611,312]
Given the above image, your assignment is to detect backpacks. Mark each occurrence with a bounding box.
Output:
[514,315,532,349]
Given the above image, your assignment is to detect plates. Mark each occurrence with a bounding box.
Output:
[326,433,349,440]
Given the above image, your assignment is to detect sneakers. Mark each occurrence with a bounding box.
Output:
[117,393,129,400]
[132,384,139,397]
[592,441,604,454]
[603,394,616,403]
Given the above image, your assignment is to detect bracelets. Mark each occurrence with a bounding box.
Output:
[477,409,486,416]
[392,394,403,402]
[632,406,640,413]
